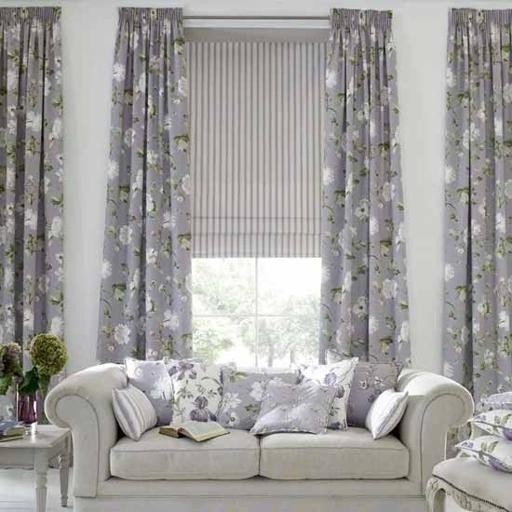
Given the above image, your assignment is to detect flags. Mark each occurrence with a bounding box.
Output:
[15,386,37,427]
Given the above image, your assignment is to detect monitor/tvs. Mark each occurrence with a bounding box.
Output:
[0,421,26,441]
[158,420,229,442]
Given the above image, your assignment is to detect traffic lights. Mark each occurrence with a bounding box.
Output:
[164,358,236,426]
[250,378,338,435]
[124,357,203,424]
[455,436,512,473]
[481,391,511,411]
[346,360,401,428]
[112,384,157,438]
[215,364,299,429]
[365,387,408,440]
[295,357,359,430]
[467,409,512,442]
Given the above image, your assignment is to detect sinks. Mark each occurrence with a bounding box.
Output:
[425,456,512,512]
[44,363,475,512]
